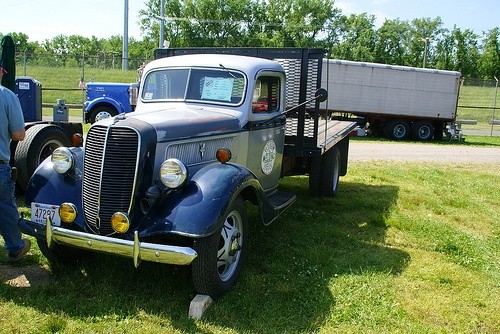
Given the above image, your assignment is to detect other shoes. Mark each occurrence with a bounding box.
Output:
[7,238,31,263]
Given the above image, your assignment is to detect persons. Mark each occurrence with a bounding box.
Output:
[137,65,144,82]
[0,66,31,261]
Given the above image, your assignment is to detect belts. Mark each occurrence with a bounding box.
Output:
[0,160,5,164]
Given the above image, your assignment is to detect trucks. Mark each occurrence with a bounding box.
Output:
[16,44,359,300]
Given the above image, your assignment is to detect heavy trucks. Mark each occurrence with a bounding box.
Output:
[82,56,463,142]
